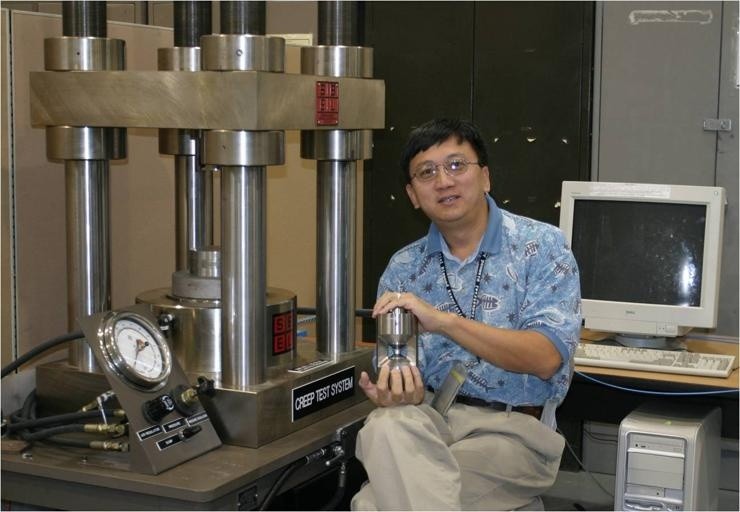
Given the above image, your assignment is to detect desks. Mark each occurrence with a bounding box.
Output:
[573,328,739,399]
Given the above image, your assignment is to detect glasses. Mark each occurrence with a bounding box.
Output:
[406,157,481,184]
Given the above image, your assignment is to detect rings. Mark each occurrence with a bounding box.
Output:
[396,292,401,299]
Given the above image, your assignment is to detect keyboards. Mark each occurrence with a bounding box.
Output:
[573,342,737,378]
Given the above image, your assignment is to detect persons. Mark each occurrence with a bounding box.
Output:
[349,118,582,511]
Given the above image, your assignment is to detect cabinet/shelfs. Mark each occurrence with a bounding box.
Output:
[590,1,739,336]
[357,2,594,343]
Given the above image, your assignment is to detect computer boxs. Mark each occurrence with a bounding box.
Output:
[614,400,723,510]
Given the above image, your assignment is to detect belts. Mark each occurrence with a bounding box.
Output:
[427,386,544,421]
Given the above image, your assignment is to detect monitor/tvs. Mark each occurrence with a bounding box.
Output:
[558,178,728,347]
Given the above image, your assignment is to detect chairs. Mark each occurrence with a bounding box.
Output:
[358,479,545,512]
